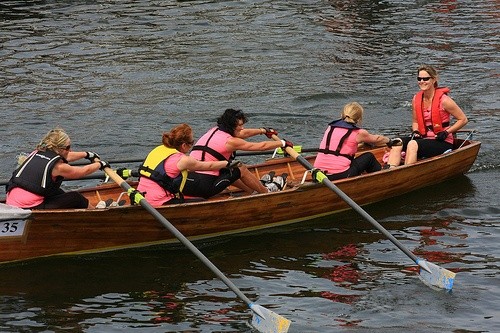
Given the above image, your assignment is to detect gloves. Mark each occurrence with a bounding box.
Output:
[280,138,292,156]
[411,130,423,140]
[99,160,112,170]
[85,151,100,163]
[387,138,402,149]
[264,127,278,139]
[435,130,448,141]
[227,158,242,168]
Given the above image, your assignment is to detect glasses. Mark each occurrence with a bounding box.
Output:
[58,145,71,151]
[417,76,432,81]
[187,140,195,146]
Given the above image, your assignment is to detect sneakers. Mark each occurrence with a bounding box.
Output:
[259,173,270,186]
[266,176,283,193]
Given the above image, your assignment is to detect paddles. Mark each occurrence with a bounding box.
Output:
[0,170,138,186]
[91,156,292,333]
[236,147,319,156]
[272,133,458,295]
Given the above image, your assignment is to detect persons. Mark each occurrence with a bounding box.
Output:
[5,130,118,210]
[388,66,468,169]
[137,124,243,206]
[313,102,401,180]
[188,108,294,197]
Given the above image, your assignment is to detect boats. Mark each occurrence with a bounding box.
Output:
[0,126,482,265]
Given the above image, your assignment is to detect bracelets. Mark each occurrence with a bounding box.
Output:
[260,128,264,135]
[281,140,285,147]
